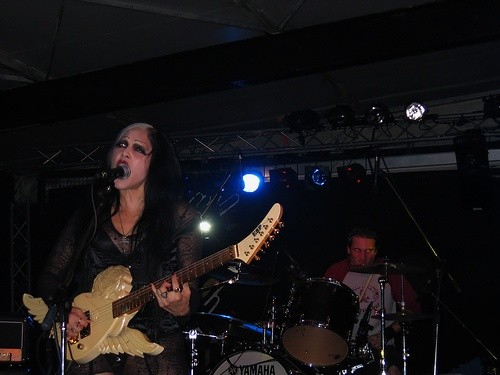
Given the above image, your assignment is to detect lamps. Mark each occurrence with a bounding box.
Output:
[366,103,390,126]
[453,128,490,177]
[326,104,353,128]
[287,109,319,131]
[404,100,429,122]
[240,164,365,193]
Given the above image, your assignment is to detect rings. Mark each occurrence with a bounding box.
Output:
[161,290,169,298]
[72,327,76,333]
[77,317,82,326]
[173,288,180,292]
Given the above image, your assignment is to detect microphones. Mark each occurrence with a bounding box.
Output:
[93,163,131,180]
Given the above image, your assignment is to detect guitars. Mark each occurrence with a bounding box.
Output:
[23,202,285,364]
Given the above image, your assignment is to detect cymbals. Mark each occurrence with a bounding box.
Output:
[192,312,273,341]
[348,261,420,275]
[372,309,426,322]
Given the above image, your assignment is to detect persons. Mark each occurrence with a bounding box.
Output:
[324,228,422,375]
[31,123,203,375]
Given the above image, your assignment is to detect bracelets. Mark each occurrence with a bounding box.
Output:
[385,324,397,338]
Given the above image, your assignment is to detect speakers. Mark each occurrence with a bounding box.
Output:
[0,320,35,375]
[454,130,489,176]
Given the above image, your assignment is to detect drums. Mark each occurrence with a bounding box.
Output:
[278,275,361,367]
[203,345,303,375]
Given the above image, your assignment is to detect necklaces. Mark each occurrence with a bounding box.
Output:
[118,207,138,253]
